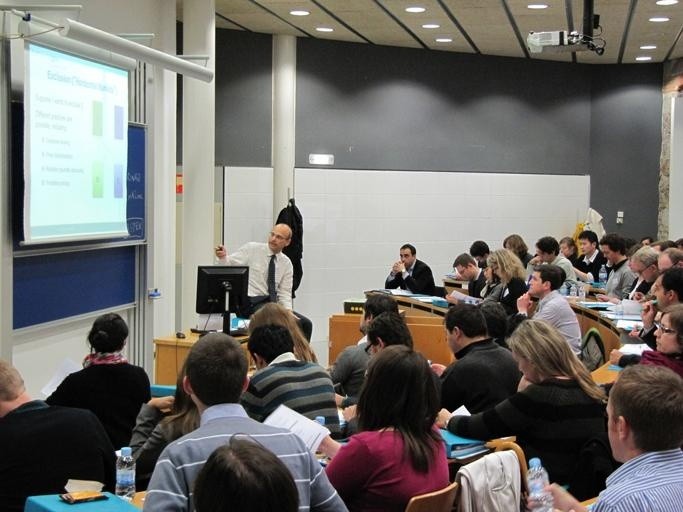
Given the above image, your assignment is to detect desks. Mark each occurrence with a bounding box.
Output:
[153,328,258,388]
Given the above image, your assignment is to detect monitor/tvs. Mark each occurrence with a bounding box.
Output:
[197,266,250,337]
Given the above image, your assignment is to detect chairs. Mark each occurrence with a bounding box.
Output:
[405,481,458,512]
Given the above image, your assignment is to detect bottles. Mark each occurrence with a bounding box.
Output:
[579,285,585,298]
[561,283,567,297]
[599,265,607,289]
[315,416,327,464]
[570,284,577,297]
[614,300,624,329]
[527,457,553,511]
[230,313,238,331]
[115,447,135,502]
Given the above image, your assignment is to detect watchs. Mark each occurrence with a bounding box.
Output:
[401,268,407,274]
[444,419,450,430]
[444,419,450,429]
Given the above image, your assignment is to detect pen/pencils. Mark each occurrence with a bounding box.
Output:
[334,382,341,388]
[638,328,644,337]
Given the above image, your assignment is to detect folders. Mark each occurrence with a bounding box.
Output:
[433,300,451,308]
[440,429,486,459]
[232,317,246,328]
[151,384,177,397]
[25,492,140,511]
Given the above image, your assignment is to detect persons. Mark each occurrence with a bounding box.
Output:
[445,258,504,310]
[193,432,300,512]
[384,243,436,296]
[0,357,118,512]
[432,318,624,503]
[452,253,487,300]
[557,236,579,264]
[605,237,637,281]
[317,344,451,512]
[430,302,523,416]
[214,223,313,343]
[247,301,318,365]
[649,241,669,253]
[524,363,683,512]
[595,244,655,306]
[469,241,493,264]
[485,248,529,319]
[515,264,582,362]
[502,234,536,270]
[44,312,160,493]
[640,236,654,247]
[238,323,344,443]
[597,304,683,433]
[653,247,683,323]
[570,230,608,296]
[480,300,513,353]
[142,332,351,512]
[630,245,661,306]
[326,293,399,397]
[526,236,577,295]
[599,232,636,301]
[610,267,683,369]
[334,310,414,439]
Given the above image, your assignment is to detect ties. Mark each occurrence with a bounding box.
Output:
[268,251,277,304]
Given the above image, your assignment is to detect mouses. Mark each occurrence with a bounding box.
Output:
[177,333,186,338]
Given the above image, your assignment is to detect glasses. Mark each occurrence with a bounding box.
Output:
[659,260,679,275]
[364,342,376,356]
[658,322,680,335]
[268,231,289,241]
[638,262,656,275]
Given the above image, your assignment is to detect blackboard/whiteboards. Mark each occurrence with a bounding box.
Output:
[9,125,149,258]
[12,243,140,330]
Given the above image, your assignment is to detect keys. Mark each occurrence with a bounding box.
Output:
[128,358,201,476]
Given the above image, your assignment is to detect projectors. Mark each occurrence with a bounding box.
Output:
[528,30,606,56]
